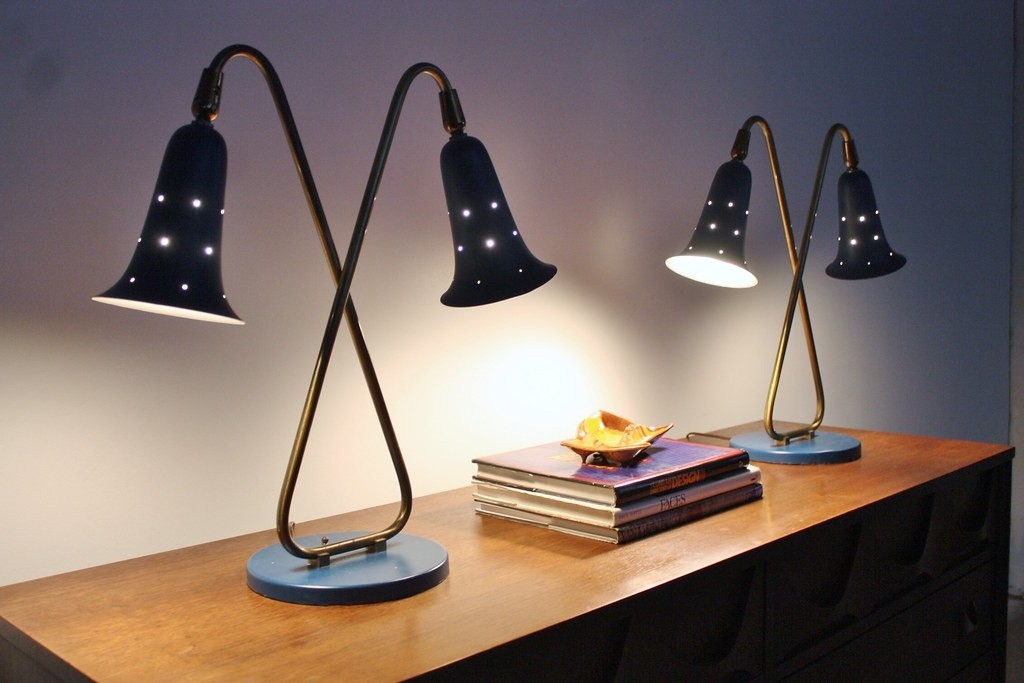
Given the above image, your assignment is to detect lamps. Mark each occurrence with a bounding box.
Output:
[665,114,908,465]
[91,44,558,607]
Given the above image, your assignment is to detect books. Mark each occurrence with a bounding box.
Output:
[470,436,763,545]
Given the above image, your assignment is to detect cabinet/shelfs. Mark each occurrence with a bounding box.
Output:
[0,418,1018,683]
